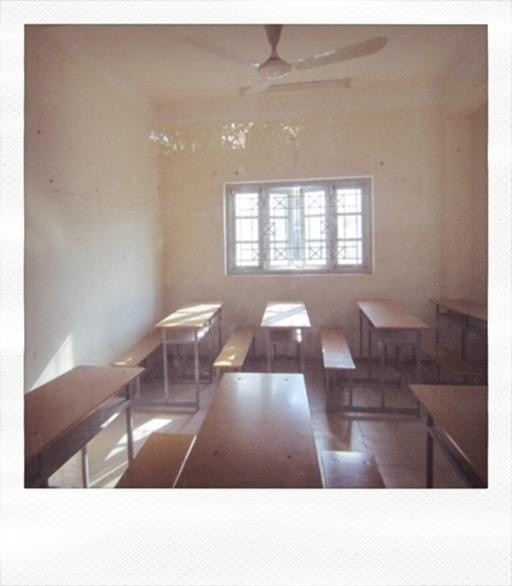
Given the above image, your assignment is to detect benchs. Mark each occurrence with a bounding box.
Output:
[213,322,256,376]
[319,327,358,413]
[115,430,195,490]
[321,451,386,487]
[417,331,465,387]
[110,328,181,409]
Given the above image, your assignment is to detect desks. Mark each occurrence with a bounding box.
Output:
[23,364,146,488]
[435,301,489,364]
[260,300,313,373]
[155,300,225,409]
[409,382,487,488]
[170,370,324,489]
[356,297,434,419]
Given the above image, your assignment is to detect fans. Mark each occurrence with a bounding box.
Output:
[179,24,388,97]
